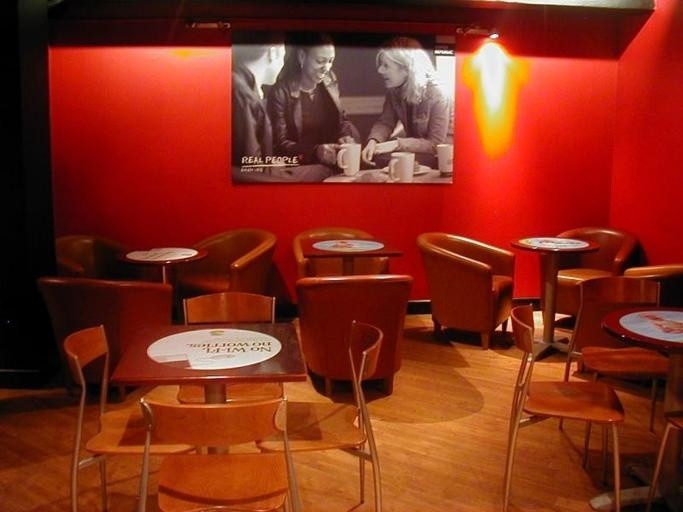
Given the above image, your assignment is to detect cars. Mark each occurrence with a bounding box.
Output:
[436,142,455,173]
[390,151,414,183]
[336,142,361,176]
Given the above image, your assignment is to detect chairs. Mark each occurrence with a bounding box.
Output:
[39,228,683,512]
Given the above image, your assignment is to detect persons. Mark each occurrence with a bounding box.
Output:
[233,44,332,182]
[362,35,454,171]
[268,44,359,169]
